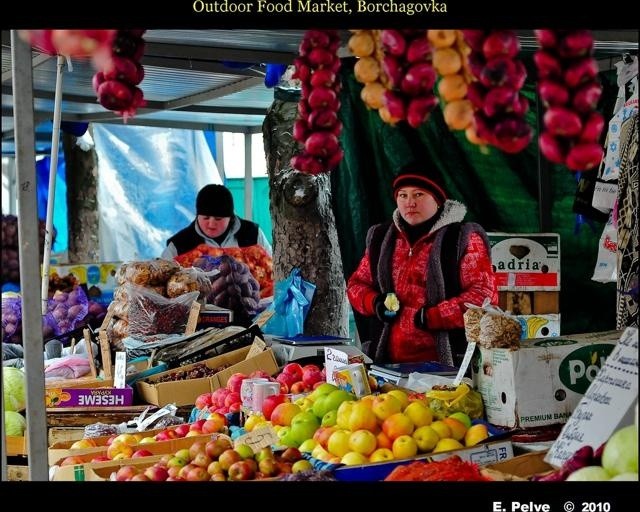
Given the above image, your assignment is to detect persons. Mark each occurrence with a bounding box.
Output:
[161,185,272,263]
[346,161,499,365]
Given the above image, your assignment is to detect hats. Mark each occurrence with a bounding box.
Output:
[195,183,235,218]
[392,159,448,205]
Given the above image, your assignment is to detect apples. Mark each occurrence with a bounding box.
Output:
[60,362,488,482]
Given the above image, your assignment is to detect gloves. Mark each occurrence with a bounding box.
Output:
[374,293,400,322]
[415,308,428,330]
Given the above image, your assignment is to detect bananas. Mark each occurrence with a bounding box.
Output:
[40,263,116,284]
[527,316,549,339]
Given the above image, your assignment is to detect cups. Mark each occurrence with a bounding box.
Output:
[240,378,279,412]
[333,362,371,399]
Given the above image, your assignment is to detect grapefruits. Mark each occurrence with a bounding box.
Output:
[610,472,638,481]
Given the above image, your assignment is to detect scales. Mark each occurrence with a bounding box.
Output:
[367,360,466,388]
[271,335,373,366]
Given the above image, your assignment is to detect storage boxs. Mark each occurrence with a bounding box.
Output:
[486,232,562,293]
[40,261,123,291]
[470,330,628,432]
[43,344,553,481]
